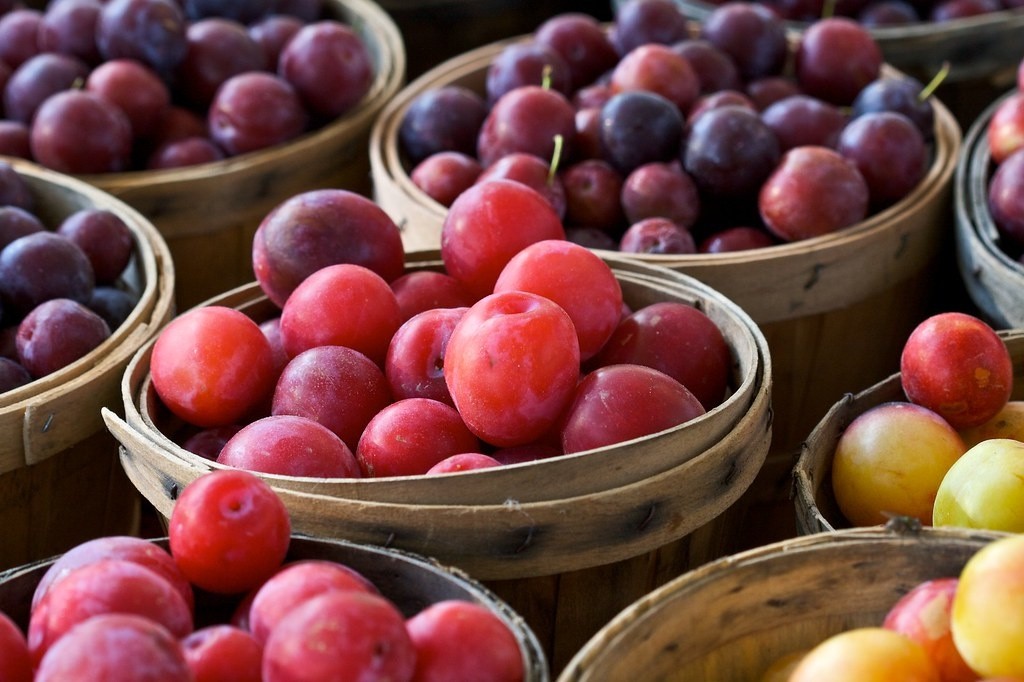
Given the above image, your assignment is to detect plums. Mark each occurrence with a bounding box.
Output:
[1,0,1024,682]
